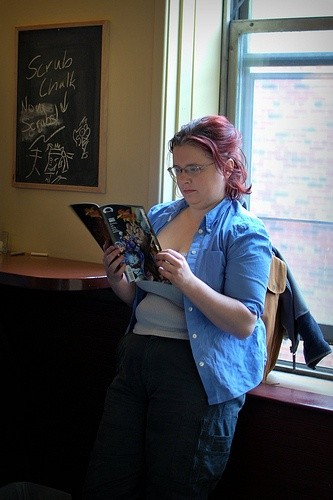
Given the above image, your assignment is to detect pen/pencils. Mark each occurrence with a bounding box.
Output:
[10,251,24,255]
[30,252,48,256]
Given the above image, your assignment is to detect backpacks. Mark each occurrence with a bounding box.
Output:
[260,252,288,385]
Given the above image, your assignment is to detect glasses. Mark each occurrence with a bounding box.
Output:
[167,161,216,177]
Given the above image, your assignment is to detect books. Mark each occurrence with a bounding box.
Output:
[68,201,171,285]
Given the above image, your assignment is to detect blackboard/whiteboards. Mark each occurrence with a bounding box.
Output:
[10,20,110,196]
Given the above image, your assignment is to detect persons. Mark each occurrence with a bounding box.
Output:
[81,114,273,499]
